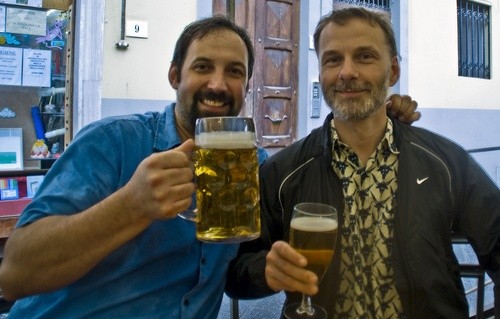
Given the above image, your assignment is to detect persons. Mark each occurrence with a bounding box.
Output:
[0,14,421,319]
[224,6,500,319]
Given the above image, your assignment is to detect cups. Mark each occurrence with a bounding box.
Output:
[177,117,261,244]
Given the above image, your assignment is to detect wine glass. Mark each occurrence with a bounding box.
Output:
[284,202,338,319]
